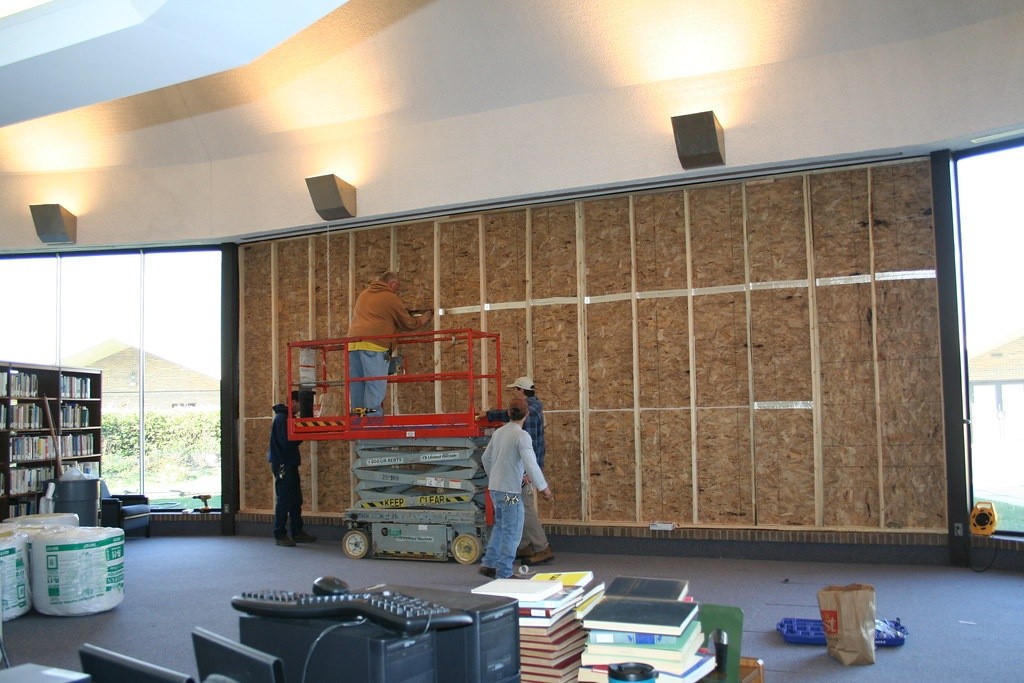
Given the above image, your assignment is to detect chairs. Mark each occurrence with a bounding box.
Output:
[100,480,151,538]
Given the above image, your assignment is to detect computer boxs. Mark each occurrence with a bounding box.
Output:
[239,584,522,683]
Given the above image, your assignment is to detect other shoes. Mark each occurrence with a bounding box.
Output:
[275,534,296,546]
[507,574,525,579]
[521,544,555,566]
[515,545,534,558]
[292,529,317,542]
[479,566,496,577]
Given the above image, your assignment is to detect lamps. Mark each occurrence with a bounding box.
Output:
[670,111,726,170]
[29,203,78,245]
[305,173,357,222]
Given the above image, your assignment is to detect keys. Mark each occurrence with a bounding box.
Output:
[384,354,389,364]
[280,470,288,481]
[269,390,317,547]
[505,496,519,507]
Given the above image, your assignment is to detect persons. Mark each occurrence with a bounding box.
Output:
[345,272,432,416]
[478,398,553,578]
[476,377,556,564]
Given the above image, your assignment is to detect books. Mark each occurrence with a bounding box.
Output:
[7,498,37,518]
[0,372,38,397]
[61,433,94,457]
[0,473,5,496]
[0,403,44,430]
[9,460,100,494]
[60,403,89,429]
[470,570,717,683]
[61,375,90,399]
[7,434,61,462]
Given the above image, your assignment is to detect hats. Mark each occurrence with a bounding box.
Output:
[508,397,529,418]
[506,377,535,390]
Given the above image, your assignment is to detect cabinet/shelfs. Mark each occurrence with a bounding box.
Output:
[0,360,102,524]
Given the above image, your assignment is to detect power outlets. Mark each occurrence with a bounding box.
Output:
[954,522,963,537]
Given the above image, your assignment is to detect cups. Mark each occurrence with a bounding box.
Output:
[607,662,659,683]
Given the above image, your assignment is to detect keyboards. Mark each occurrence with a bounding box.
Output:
[231,589,473,632]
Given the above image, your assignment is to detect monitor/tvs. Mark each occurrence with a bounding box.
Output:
[78,626,287,683]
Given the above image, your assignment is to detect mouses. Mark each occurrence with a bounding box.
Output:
[312,575,349,596]
